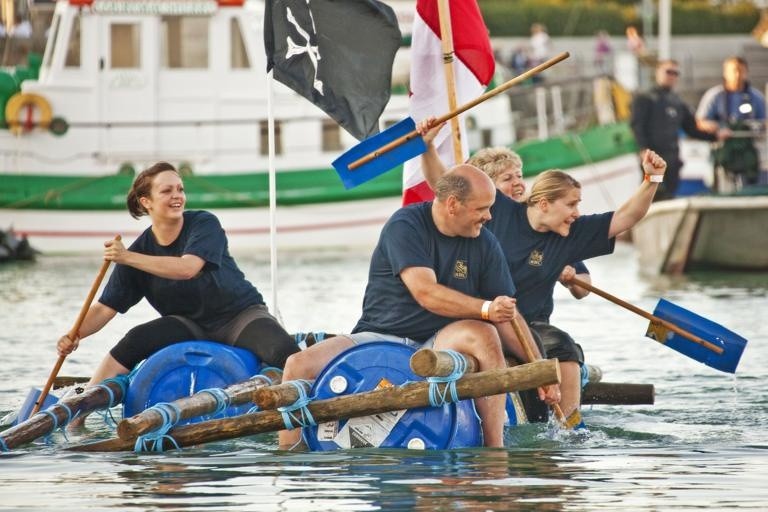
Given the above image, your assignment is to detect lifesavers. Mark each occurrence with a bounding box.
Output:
[4,95,53,136]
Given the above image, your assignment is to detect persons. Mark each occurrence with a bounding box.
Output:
[418,118,668,437]
[57,163,301,429]
[479,18,646,92]
[466,146,592,300]
[694,56,767,197]
[628,60,729,201]
[277,166,562,485]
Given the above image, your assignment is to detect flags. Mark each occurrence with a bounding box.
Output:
[264,0,401,141]
[402,0,495,208]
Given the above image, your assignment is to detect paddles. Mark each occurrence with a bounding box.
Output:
[14,231,122,429]
[561,271,748,375]
[510,316,589,430]
[331,50,570,191]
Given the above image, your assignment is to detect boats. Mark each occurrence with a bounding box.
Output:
[0,330,653,464]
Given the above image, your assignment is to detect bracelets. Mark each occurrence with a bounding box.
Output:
[481,300,492,322]
[645,175,664,185]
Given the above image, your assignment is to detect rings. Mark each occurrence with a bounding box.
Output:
[557,392,561,396]
